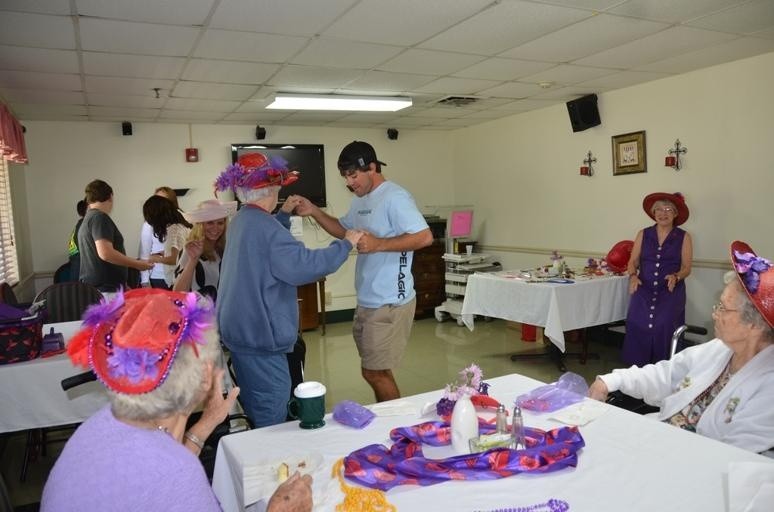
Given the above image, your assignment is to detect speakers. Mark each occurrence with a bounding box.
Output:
[566,94,600,132]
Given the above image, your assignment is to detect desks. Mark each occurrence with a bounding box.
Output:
[0,320,227,483]
[212,371,774,512]
[461,267,640,372]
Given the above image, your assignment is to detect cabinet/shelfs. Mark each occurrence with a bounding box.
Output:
[297,282,319,332]
[434,253,495,326]
[410,240,446,320]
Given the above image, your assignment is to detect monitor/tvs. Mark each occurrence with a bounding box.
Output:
[232,144,327,212]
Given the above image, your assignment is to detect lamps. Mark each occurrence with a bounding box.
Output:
[580,150,597,176]
[665,138,687,170]
[263,91,413,112]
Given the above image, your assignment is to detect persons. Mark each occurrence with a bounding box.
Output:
[137,187,178,289]
[215,153,363,430]
[37,287,314,512]
[295,141,435,401]
[142,194,194,292]
[67,197,87,273]
[618,191,693,367]
[587,241,773,459]
[76,179,155,304]
[172,200,238,302]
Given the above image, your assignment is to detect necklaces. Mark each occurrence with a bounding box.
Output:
[473,496,570,512]
[149,418,174,436]
[329,454,396,512]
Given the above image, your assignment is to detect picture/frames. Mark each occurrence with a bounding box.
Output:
[611,130,647,176]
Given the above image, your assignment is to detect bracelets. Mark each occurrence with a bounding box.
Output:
[629,272,637,280]
[673,272,681,284]
[185,430,205,448]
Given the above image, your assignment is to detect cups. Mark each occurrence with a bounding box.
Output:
[465,244,473,257]
[287,380,328,429]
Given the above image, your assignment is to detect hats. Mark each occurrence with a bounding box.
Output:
[643,193,689,225]
[337,141,387,171]
[183,199,238,225]
[214,153,298,189]
[66,287,216,396]
[606,240,635,273]
[729,241,774,328]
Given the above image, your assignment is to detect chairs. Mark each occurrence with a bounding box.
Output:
[0,282,33,310]
[31,283,106,456]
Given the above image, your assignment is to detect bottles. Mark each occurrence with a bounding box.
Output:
[511,407,527,451]
[496,403,508,435]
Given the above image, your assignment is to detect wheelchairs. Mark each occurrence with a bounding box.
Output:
[62,359,256,471]
[602,321,709,419]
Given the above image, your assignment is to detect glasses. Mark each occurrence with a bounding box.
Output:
[712,304,736,319]
[654,206,674,215]
